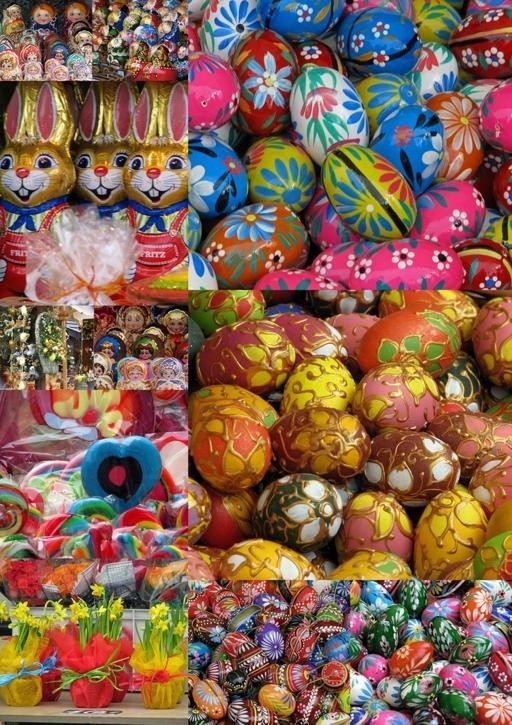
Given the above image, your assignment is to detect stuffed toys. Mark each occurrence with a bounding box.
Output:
[0,0,512,725]
